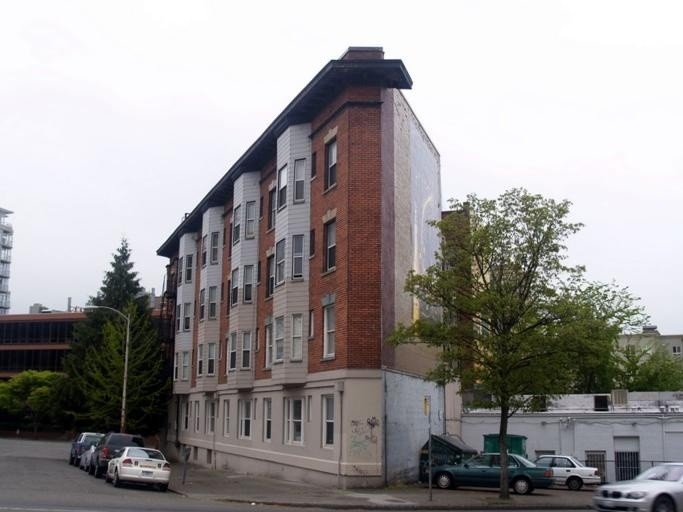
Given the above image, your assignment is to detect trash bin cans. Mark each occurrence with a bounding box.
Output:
[483,434,528,466]
[418,434,479,484]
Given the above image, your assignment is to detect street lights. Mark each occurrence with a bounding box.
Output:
[84,306,130,432]
[425,395,432,500]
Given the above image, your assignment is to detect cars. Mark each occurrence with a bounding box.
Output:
[431,452,554,494]
[70,432,171,491]
[593,463,682,511]
[530,454,601,489]
